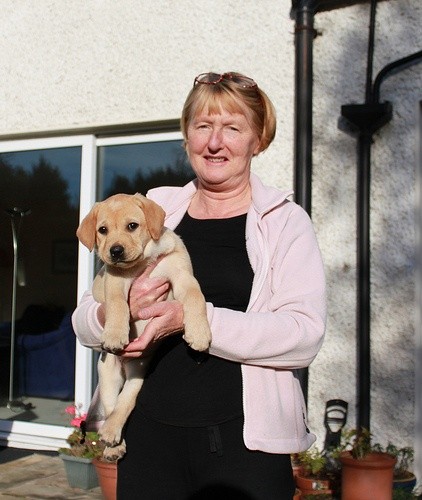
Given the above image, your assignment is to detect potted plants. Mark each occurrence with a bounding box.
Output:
[92,439,126,500]
[291,427,422,500]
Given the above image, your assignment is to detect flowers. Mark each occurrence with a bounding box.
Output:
[58,402,100,459]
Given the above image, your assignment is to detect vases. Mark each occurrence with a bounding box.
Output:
[62,456,99,491]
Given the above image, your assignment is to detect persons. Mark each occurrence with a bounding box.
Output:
[71,72,327,500]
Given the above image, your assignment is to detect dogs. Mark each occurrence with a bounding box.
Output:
[75,192,213,464]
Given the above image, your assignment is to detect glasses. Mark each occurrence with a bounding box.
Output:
[194,73,260,99]
